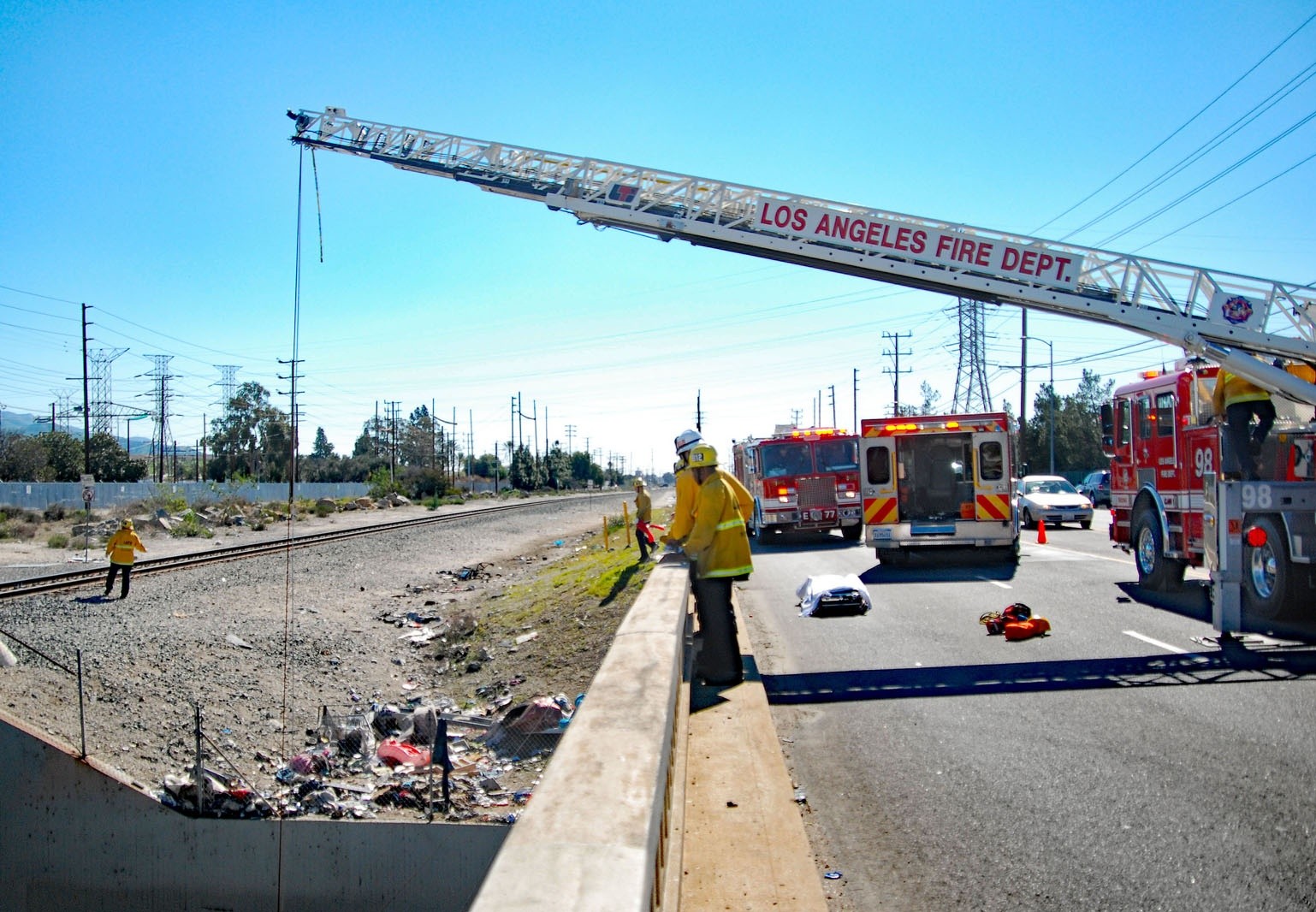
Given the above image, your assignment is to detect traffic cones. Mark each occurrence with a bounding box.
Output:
[1036,520,1049,544]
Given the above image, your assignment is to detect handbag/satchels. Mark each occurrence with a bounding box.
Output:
[980,602,1051,639]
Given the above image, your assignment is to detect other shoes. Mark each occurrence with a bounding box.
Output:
[650,545,660,553]
[693,630,743,685]
[638,554,649,561]
[1249,472,1260,480]
[1247,438,1261,456]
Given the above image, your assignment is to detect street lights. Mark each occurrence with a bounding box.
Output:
[1020,336,1054,474]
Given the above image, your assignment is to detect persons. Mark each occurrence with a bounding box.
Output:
[774,447,850,476]
[1211,347,1276,482]
[634,479,659,561]
[106,517,147,598]
[660,429,754,686]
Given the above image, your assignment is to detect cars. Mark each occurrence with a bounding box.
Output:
[1019,475,1094,529]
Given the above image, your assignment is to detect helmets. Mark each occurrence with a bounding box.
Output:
[634,478,647,487]
[677,429,707,455]
[685,443,718,468]
[121,519,132,528]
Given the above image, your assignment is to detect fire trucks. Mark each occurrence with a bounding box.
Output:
[732,423,866,546]
[287,105,1316,638]
[860,412,1028,567]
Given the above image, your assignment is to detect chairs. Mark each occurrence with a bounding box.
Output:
[1049,482,1061,493]
[868,445,956,521]
[825,448,847,467]
[765,448,806,467]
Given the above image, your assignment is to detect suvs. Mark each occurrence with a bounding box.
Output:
[1076,470,1112,509]
